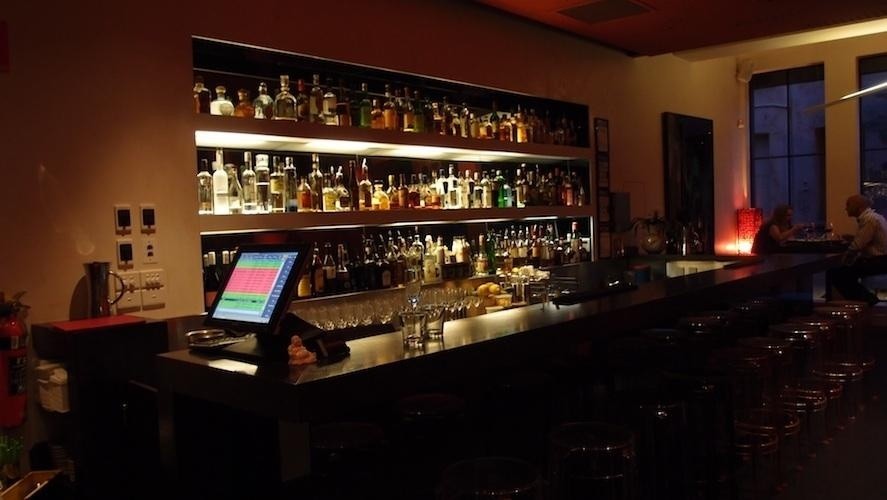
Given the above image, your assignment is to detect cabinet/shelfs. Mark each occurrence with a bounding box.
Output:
[202,36,593,315]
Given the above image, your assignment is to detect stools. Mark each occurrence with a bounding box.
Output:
[735,335,799,490]
[767,326,832,449]
[619,387,696,500]
[436,456,548,500]
[703,348,789,500]
[825,298,880,411]
[662,366,737,500]
[812,306,865,427]
[546,423,641,500]
[788,317,843,441]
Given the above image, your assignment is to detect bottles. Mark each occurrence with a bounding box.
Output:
[193,73,577,144]
[195,221,589,309]
[195,147,593,216]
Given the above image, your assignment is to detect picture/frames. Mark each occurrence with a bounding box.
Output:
[593,117,612,261]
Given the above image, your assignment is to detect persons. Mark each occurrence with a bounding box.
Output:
[287,335,316,364]
[750,204,803,248]
[831,195,887,306]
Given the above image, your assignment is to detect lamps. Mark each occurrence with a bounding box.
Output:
[733,207,763,256]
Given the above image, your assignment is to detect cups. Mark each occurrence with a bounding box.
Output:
[420,304,447,338]
[399,311,426,345]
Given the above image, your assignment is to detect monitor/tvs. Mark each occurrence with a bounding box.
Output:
[203,242,306,360]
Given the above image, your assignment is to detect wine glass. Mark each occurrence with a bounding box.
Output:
[288,286,484,334]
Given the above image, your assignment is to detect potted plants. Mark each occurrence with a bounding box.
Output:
[627,210,674,254]
